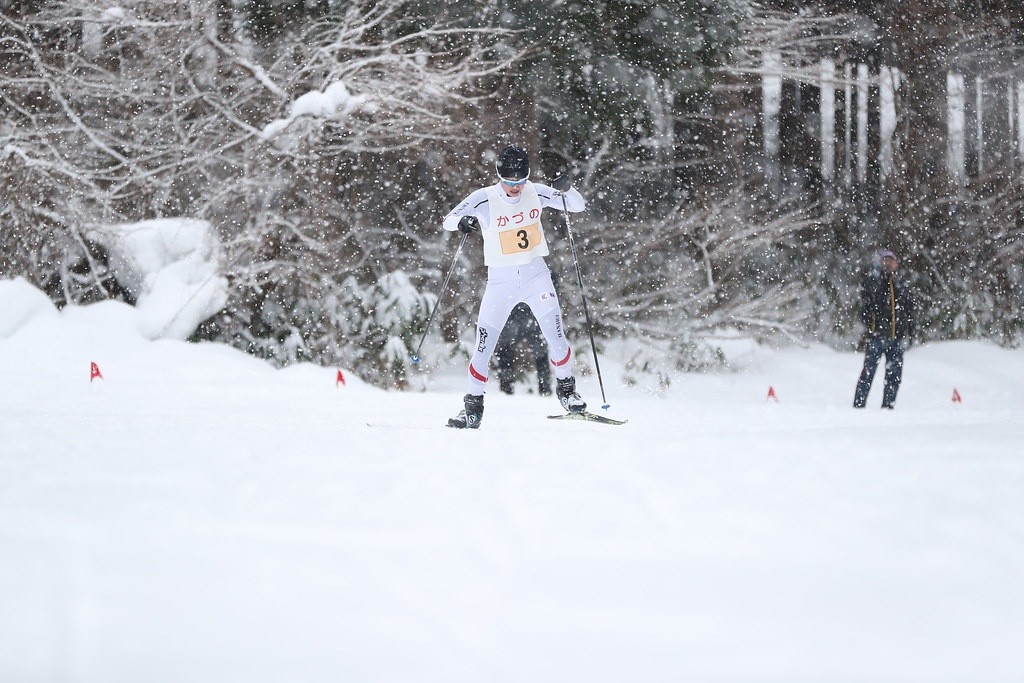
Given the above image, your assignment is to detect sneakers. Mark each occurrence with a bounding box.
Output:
[447,394,485,428]
[556,378,588,414]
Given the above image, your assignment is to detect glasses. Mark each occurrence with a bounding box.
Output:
[501,178,527,187]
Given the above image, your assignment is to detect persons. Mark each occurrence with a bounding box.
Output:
[441,146,587,429]
[853,250,915,409]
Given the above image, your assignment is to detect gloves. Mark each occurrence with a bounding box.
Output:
[458,217,478,234]
[548,174,571,192]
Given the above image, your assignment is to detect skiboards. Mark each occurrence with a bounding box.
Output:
[364,415,628,428]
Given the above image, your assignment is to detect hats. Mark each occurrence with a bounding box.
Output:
[498,147,529,178]
[881,251,897,262]
